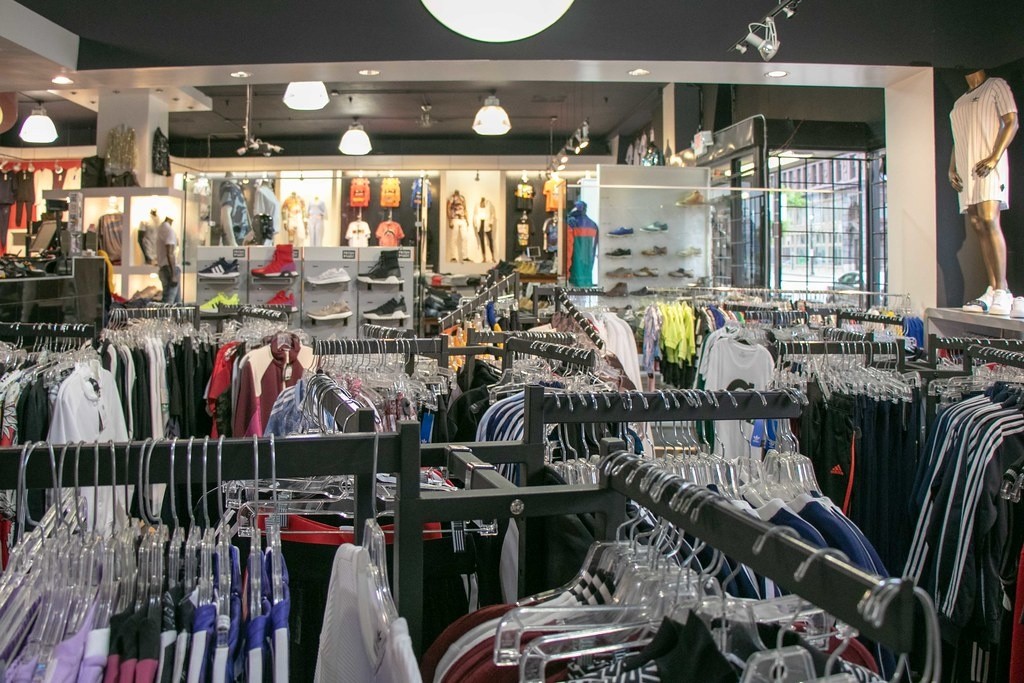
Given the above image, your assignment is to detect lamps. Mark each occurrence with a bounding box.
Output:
[471,89,512,135]
[282,81,331,111]
[546,121,589,180]
[18,102,59,144]
[338,116,374,155]
[733,6,796,61]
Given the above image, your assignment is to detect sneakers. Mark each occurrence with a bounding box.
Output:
[200,293,239,313]
[363,297,410,319]
[307,268,351,284]
[306,301,353,321]
[197,257,241,277]
[266,290,298,313]
[962,285,1024,318]
[0,256,46,278]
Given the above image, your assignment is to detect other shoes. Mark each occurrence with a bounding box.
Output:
[607,227,635,238]
[606,268,635,277]
[633,267,660,277]
[605,248,632,260]
[678,247,702,257]
[676,191,704,207]
[487,258,558,278]
[629,286,657,298]
[643,245,668,256]
[641,222,669,234]
[669,268,695,278]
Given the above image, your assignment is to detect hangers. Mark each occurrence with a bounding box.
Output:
[0,270,1024,683]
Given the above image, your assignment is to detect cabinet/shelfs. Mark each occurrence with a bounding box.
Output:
[42,187,210,303]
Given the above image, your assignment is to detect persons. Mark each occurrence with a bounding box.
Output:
[304,196,328,248]
[253,181,279,245]
[281,193,308,248]
[948,70,1019,315]
[447,190,471,261]
[474,197,496,263]
[219,172,254,246]
[567,201,598,287]
[138,209,160,266]
[156,216,183,303]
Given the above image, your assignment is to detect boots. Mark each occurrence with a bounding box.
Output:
[252,244,298,277]
[607,283,629,297]
[357,250,405,284]
